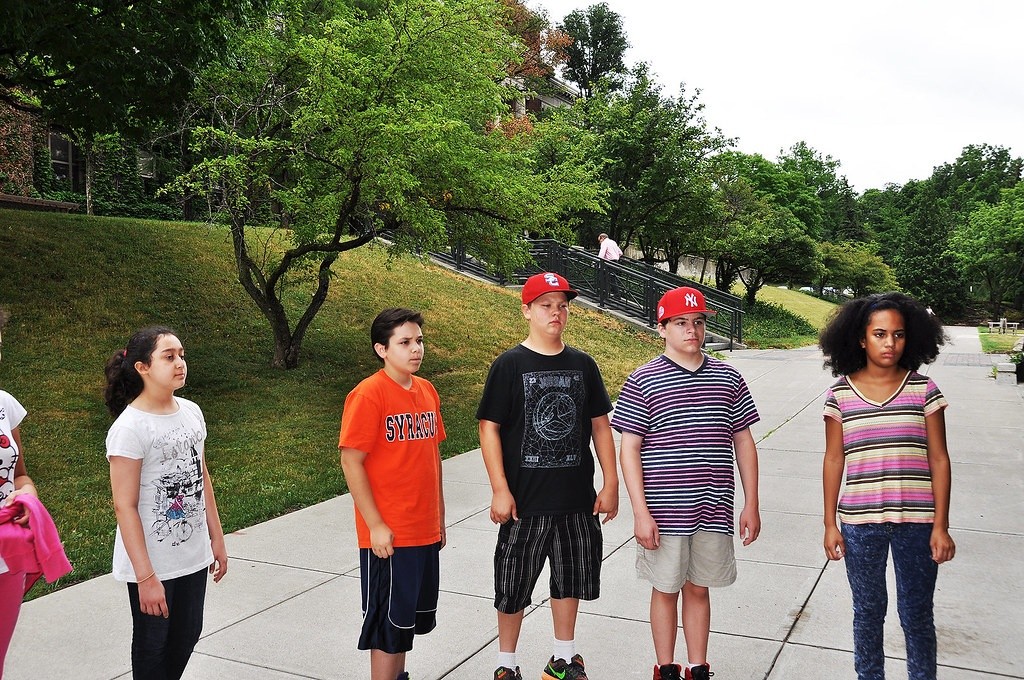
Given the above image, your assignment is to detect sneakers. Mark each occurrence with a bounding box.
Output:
[685,663,715,680]
[653,663,682,680]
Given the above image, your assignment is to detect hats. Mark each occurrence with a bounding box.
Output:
[522,272,579,305]
[656,287,717,323]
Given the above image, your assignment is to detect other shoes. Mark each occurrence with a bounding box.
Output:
[541,655,589,680]
[494,666,522,680]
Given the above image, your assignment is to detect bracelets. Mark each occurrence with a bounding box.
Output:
[138,571,155,584]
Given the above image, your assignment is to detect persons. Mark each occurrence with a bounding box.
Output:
[105,325,228,680]
[819,292,956,680]
[0,330,74,680]
[476,272,620,680]
[338,307,448,680]
[595,232,623,308]
[608,286,760,680]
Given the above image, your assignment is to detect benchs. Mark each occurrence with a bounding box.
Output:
[988,318,1019,335]
[992,354,1017,384]
